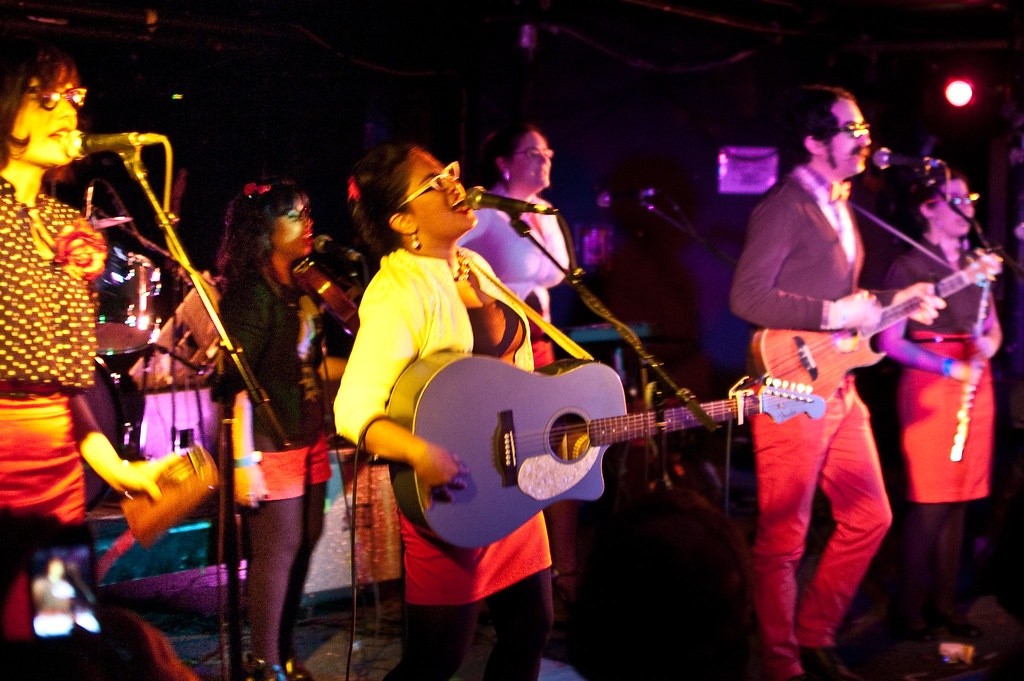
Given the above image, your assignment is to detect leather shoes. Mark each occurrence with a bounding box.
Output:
[799,646,863,681]
[932,610,981,637]
[249,664,285,681]
[284,657,312,681]
[895,610,935,641]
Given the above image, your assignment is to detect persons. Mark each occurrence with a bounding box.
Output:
[877,156,1004,641]
[32,558,75,635]
[731,87,946,681]
[331,143,554,681]
[216,173,331,681]
[470,123,579,365]
[1,23,207,646]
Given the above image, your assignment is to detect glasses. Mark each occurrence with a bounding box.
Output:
[515,147,554,159]
[817,122,871,136]
[928,196,974,207]
[24,87,87,111]
[396,161,460,211]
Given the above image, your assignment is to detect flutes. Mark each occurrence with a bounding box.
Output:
[950,277,992,464]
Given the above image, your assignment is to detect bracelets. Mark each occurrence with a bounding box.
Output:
[940,356,956,376]
[232,451,262,467]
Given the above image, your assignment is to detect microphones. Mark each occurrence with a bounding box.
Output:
[466,185,559,217]
[314,235,364,261]
[64,130,165,161]
[874,147,947,170]
[599,188,656,207]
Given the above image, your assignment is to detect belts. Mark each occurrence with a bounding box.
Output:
[911,338,967,342]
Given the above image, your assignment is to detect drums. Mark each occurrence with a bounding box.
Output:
[79,357,125,512]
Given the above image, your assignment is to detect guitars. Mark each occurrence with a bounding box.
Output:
[749,248,1002,402]
[385,350,827,549]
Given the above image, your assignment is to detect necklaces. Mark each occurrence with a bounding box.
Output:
[451,247,470,281]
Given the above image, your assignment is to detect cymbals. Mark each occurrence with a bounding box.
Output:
[91,320,151,356]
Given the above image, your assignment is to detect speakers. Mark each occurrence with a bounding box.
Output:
[297,449,406,609]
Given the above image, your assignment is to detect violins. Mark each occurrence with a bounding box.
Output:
[298,261,359,324]
